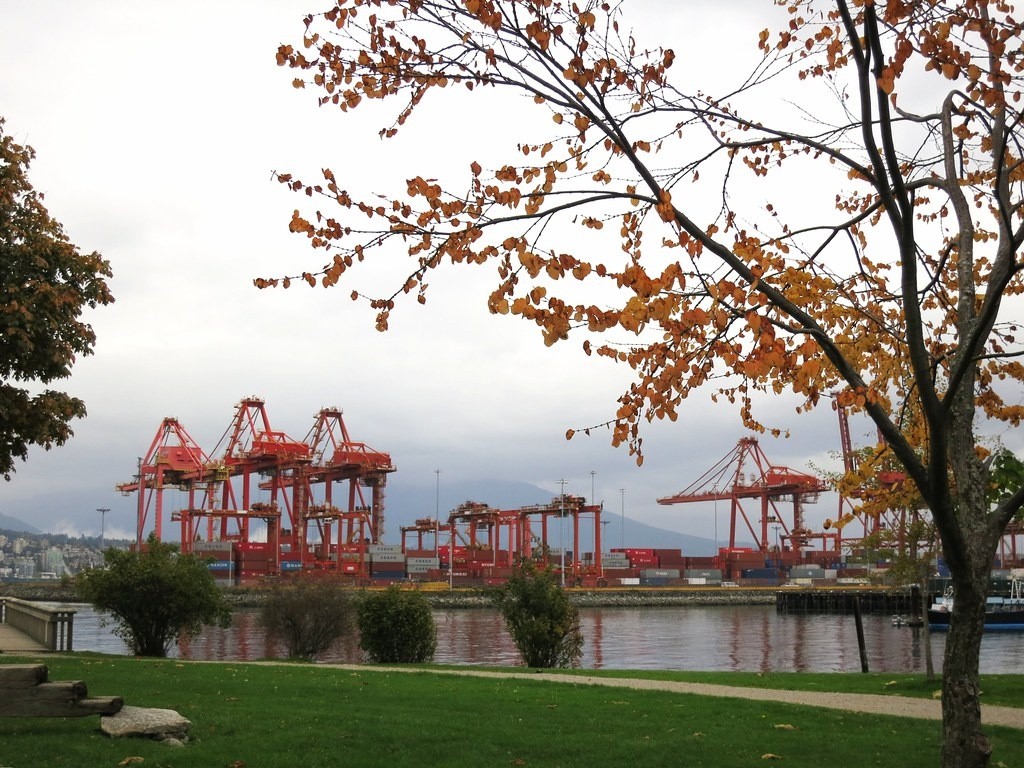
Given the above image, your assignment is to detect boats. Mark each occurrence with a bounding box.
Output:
[922,568,1024,626]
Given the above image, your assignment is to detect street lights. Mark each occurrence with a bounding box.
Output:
[590,470,597,566]
[436,468,441,567]
[600,520,612,556]
[555,478,569,589]
[772,526,781,554]
[713,482,719,553]
[95,507,112,552]
[620,489,628,549]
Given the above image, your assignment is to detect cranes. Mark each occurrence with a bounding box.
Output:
[656,436,843,587]
[399,492,608,588]
[116,394,397,585]
